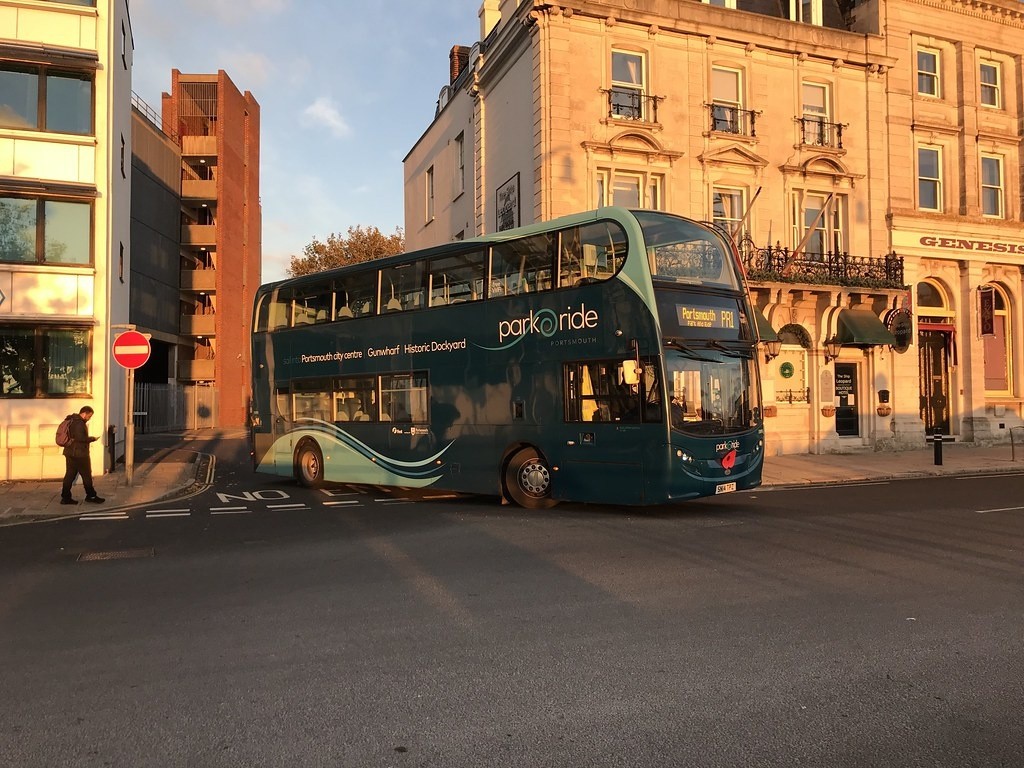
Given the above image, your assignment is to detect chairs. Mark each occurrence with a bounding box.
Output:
[337,306,353,320]
[414,293,425,310]
[295,314,309,327]
[406,299,415,310]
[361,301,374,316]
[387,298,402,311]
[431,297,446,306]
[315,310,329,323]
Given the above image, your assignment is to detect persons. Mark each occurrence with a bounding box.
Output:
[620,372,643,410]
[60,406,106,505]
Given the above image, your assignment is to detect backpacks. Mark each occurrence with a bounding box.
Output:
[56,413,86,447]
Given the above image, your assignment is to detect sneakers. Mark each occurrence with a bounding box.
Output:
[85,495,105,504]
[60,498,79,505]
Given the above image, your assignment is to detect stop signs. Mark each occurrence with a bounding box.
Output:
[112,331,151,370]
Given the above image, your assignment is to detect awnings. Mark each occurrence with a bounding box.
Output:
[753,305,955,345]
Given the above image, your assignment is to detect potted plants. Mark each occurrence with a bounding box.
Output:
[877,404,892,417]
[822,405,837,417]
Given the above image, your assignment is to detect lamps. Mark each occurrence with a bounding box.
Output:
[825,333,843,364]
[765,336,785,365]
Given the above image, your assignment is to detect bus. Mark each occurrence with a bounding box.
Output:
[251,206,766,510]
